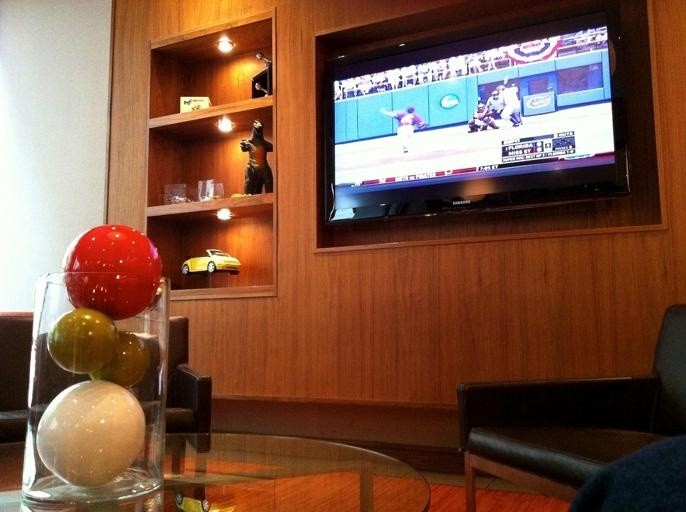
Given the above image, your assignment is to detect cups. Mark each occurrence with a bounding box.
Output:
[22,269,166,501]
[163,177,225,207]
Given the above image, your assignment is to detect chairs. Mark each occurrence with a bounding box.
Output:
[1,310,213,458]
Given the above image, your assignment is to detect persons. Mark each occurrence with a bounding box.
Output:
[380,104,427,153]
[487,90,504,113]
[467,101,499,133]
[496,85,524,127]
[333,26,608,103]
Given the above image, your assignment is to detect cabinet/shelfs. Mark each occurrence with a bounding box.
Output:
[144,10,277,299]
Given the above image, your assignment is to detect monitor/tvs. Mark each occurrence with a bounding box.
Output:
[318,10,631,228]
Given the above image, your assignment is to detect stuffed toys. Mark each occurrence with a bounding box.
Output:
[240,119,274,195]
